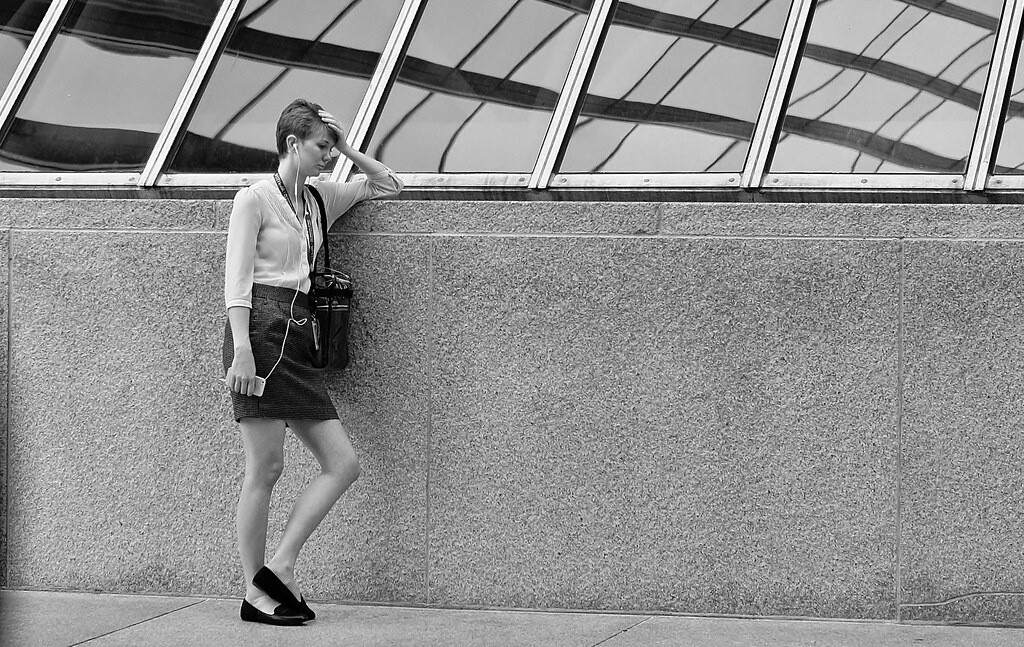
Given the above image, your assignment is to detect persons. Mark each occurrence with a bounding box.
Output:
[222,98,404,625]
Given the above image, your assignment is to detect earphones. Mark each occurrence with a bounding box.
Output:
[293,143,298,153]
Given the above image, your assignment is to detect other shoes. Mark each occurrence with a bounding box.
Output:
[240,598,305,626]
[252,566,315,620]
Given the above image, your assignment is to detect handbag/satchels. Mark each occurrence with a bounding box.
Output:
[307,282,353,372]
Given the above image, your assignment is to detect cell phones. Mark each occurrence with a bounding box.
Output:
[225,367,267,397]
